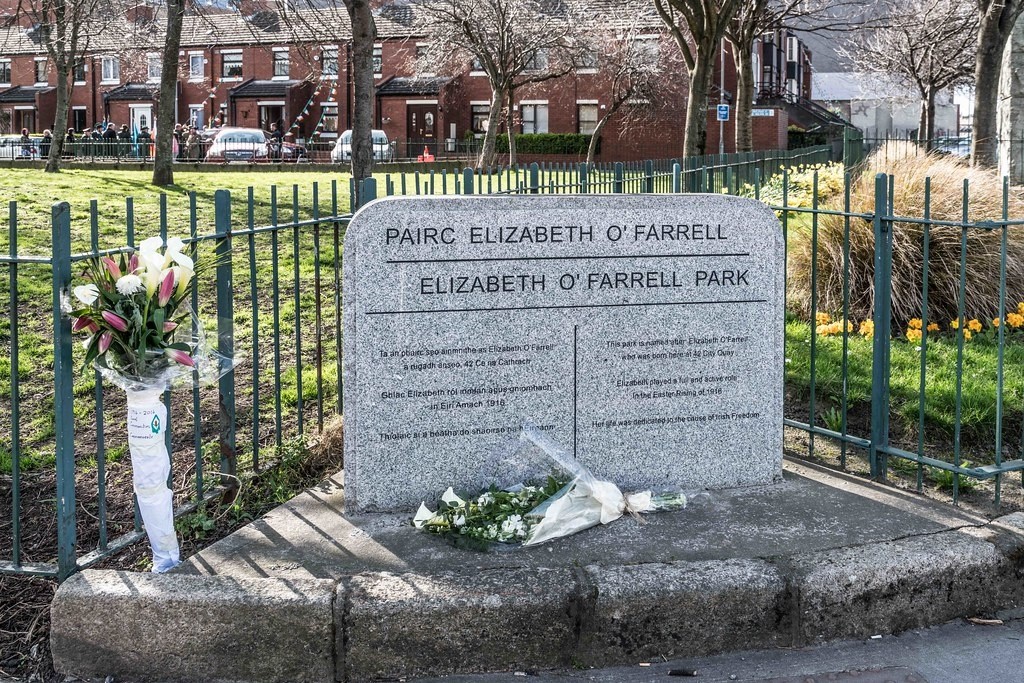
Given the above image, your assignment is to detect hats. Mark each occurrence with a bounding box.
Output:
[142,126,149,129]
[83,128,90,132]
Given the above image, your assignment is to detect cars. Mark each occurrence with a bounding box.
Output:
[328,129,396,163]
[927,122,972,161]
[201,128,309,161]
[204,126,273,163]
[0,137,41,159]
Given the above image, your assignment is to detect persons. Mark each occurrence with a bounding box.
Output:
[270,123,282,163]
[21,123,208,163]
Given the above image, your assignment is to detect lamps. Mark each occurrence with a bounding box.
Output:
[34,104,38,111]
[439,104,443,112]
[150,106,155,113]
[513,104,519,113]
[600,103,606,112]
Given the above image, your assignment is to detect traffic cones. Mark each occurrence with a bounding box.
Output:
[423,146,431,157]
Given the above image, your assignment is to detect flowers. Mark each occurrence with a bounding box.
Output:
[400,466,688,555]
[67,230,234,391]
